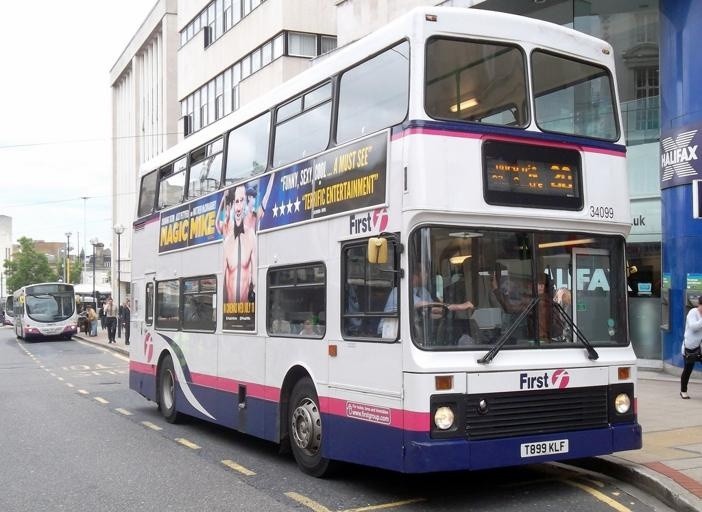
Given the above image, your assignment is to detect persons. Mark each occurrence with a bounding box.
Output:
[79,293,130,346]
[299,313,319,336]
[679,295,701,400]
[216,170,279,324]
[377,258,474,338]
[443,257,572,345]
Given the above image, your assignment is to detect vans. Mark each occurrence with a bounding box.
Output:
[78,296,105,319]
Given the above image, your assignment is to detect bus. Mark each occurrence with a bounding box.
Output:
[3,294,15,325]
[127,5,646,480]
[12,280,79,340]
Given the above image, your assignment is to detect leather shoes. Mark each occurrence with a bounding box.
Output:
[680,392,690,399]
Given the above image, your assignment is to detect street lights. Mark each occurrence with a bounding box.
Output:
[64,231,74,258]
[114,221,126,339]
[89,235,99,302]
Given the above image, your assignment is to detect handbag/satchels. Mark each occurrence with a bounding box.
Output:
[684,348,701,360]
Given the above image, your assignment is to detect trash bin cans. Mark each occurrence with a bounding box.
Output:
[79,317,86,332]
